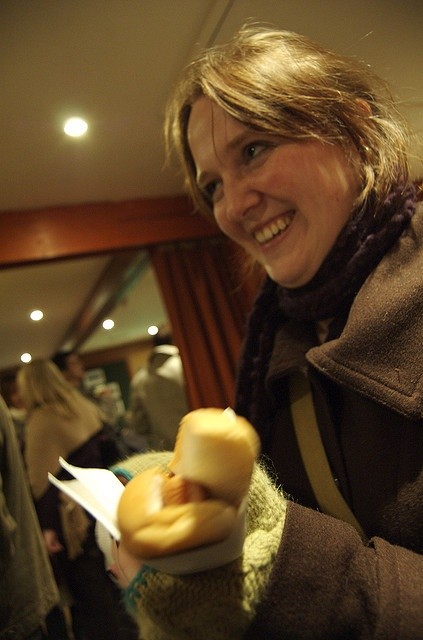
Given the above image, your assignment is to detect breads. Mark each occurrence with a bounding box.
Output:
[172,406,261,500]
[117,468,236,560]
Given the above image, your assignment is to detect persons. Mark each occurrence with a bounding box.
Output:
[119,330,192,448]
[0,389,61,639]
[1,365,25,460]
[15,351,139,638]
[52,351,112,406]
[93,18,422,638]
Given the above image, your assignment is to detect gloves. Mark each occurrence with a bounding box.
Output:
[88,450,288,638]
